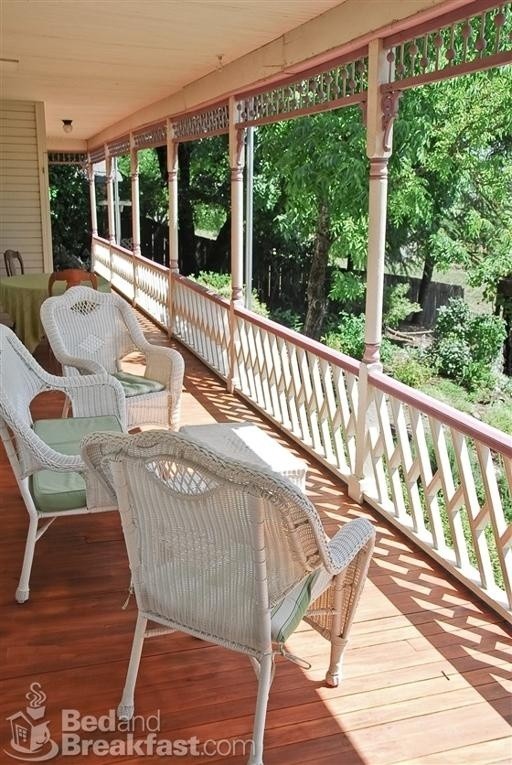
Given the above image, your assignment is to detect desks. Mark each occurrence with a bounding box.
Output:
[179,420,308,496]
[1,272,111,355]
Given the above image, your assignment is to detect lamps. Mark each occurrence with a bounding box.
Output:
[61,120,72,134]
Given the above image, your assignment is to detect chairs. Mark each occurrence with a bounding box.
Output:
[3,248,23,278]
[39,287,185,434]
[43,268,98,368]
[77,423,378,764]
[1,320,148,606]
